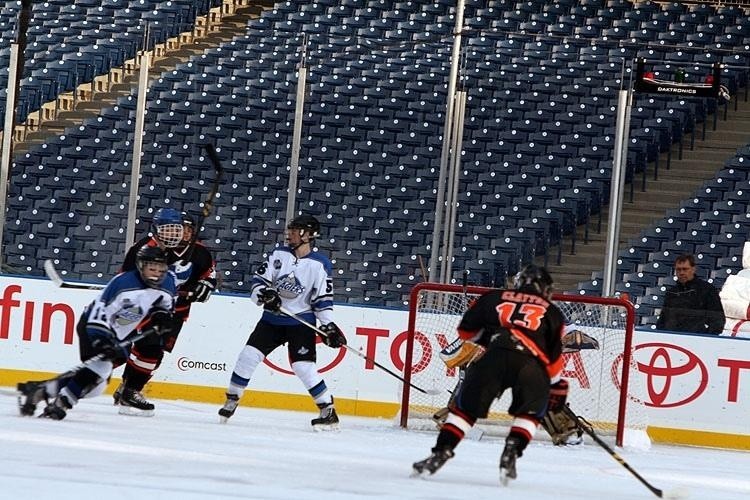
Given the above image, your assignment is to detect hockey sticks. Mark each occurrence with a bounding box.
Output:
[563,404,690,498]
[182,142,223,264]
[276,301,439,396]
[46,327,160,396]
[43,259,105,290]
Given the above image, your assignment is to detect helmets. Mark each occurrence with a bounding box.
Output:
[513,261,555,298]
[178,210,197,247]
[287,213,323,243]
[148,206,185,248]
[134,244,169,288]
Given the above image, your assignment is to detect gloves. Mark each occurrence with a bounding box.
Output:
[93,337,127,369]
[317,321,348,349]
[255,286,283,315]
[548,380,569,416]
[147,306,179,334]
[187,276,216,304]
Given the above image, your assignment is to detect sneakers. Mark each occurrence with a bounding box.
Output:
[44,393,73,421]
[218,392,241,418]
[22,378,48,417]
[557,429,584,445]
[412,449,455,477]
[310,394,340,425]
[111,382,157,411]
[499,441,519,479]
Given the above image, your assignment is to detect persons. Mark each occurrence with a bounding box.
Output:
[408,262,564,484]
[217,214,346,426]
[120,207,184,273]
[14,243,176,421]
[112,209,215,417]
[652,253,727,336]
